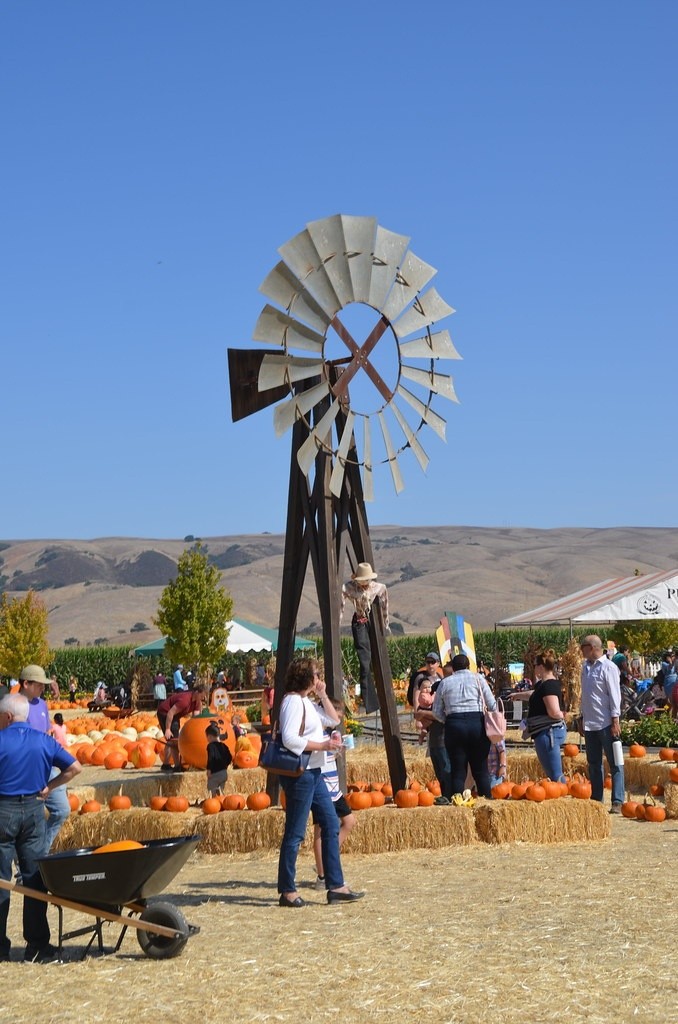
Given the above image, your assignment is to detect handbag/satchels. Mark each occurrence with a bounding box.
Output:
[482,697,507,743]
[257,733,313,776]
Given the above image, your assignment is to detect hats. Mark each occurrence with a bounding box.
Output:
[424,652,439,661]
[353,562,377,581]
[19,664,54,684]
[69,654,277,690]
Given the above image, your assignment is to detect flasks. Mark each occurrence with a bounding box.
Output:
[612,735,624,767]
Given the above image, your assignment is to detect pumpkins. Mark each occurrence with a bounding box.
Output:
[11,684,678,854]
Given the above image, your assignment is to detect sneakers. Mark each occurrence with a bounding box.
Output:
[315,875,325,889]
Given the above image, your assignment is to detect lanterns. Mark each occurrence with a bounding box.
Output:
[178,710,237,771]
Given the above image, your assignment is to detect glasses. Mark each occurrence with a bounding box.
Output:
[314,672,321,680]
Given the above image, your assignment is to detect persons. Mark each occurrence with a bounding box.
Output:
[48,713,68,748]
[340,562,391,715]
[0,692,67,965]
[206,722,232,797]
[408,635,678,814]
[0,659,272,709]
[275,658,368,908]
[13,665,73,886]
[156,685,207,772]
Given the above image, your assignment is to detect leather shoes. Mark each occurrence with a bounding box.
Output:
[327,887,366,904]
[277,893,305,907]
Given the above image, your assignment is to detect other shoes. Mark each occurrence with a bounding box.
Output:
[608,802,623,813]
[24,943,64,962]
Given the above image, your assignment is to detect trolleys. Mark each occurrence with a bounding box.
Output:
[0,835,205,959]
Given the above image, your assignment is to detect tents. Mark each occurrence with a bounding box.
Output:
[134,617,317,657]
[493,568,678,660]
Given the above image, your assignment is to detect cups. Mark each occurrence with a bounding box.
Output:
[342,733,354,750]
